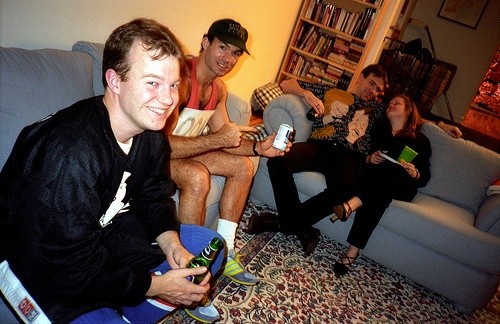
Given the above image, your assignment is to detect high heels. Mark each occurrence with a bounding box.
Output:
[333,249,360,275]
[329,201,353,223]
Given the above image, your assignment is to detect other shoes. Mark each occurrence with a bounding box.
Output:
[296,227,321,256]
[222,250,260,286]
[183,298,220,323]
[248,212,281,235]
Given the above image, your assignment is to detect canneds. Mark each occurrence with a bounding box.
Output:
[272,124,293,151]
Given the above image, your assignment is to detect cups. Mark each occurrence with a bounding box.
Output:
[398,145,418,163]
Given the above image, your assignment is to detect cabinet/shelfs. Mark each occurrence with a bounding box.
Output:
[277,0,385,101]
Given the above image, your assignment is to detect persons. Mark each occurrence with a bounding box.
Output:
[330,95,431,277]
[0,19,225,324]
[165,19,291,324]
[248,64,462,258]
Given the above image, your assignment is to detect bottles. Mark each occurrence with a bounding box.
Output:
[184,237,222,285]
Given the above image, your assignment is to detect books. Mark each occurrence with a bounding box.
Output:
[283,0,384,101]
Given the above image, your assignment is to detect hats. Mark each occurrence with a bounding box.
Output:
[207,18,251,57]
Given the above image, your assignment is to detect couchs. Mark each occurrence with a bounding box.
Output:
[246,93,500,315]
[0,41,260,324]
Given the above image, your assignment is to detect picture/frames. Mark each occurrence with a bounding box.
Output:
[437,0,488,31]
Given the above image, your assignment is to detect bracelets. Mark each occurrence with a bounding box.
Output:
[415,169,418,178]
[253,141,258,155]
[436,120,440,125]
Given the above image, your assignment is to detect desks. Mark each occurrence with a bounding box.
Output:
[378,46,458,118]
